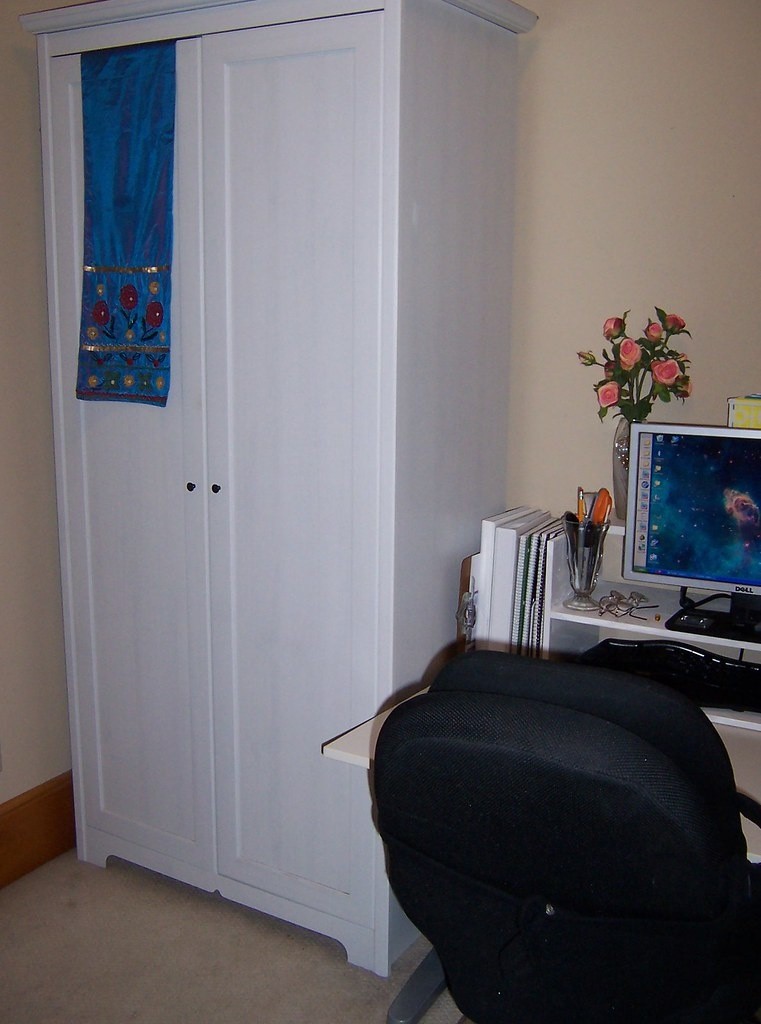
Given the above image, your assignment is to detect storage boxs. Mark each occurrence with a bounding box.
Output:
[727,396,761,431]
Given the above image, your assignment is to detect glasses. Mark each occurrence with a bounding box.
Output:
[597,590,660,621]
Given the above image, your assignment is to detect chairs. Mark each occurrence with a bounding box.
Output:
[375,649,761,1024]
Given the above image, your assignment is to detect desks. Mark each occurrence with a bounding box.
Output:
[322,681,761,1024]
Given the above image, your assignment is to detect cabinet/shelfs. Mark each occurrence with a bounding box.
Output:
[17,1,540,977]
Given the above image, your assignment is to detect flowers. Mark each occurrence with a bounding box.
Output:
[576,306,692,426]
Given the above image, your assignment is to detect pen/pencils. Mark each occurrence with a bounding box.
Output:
[563,487,613,591]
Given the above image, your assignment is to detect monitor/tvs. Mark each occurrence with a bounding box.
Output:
[623,423,761,646]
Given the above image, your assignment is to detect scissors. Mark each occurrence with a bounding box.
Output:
[587,489,612,542]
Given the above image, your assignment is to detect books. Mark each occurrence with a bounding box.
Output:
[465,505,563,659]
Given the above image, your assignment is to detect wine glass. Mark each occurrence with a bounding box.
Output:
[561,518,611,611]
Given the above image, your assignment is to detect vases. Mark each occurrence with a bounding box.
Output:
[614,418,647,519]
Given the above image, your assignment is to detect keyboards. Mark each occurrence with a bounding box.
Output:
[580,639,761,713]
